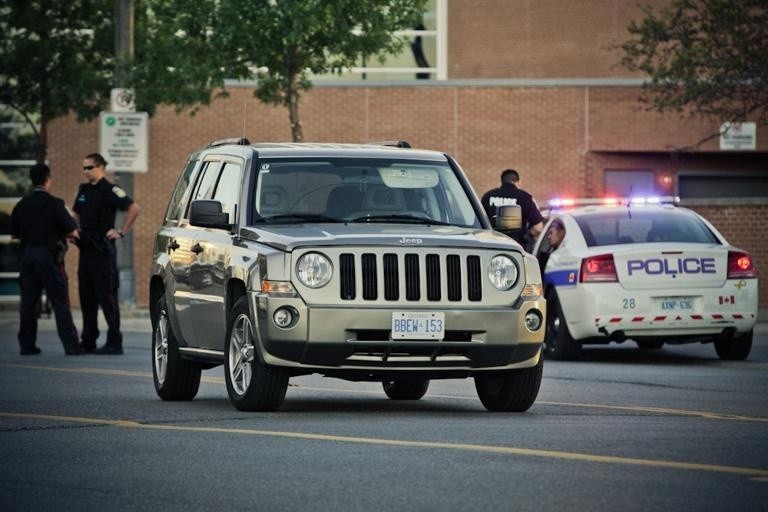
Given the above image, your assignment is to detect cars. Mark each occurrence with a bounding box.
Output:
[527,187,762,363]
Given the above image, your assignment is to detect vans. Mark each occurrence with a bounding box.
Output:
[0,160,51,271]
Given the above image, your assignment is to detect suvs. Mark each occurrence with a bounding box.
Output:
[143,134,546,425]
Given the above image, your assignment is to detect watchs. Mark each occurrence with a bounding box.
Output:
[116,227,124,240]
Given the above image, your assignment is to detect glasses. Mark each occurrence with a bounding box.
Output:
[83,163,99,170]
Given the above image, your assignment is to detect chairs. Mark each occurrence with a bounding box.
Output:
[260,185,288,219]
[349,186,407,220]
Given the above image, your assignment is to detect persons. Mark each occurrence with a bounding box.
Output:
[544,221,563,263]
[480,170,544,251]
[11,161,87,356]
[67,153,141,356]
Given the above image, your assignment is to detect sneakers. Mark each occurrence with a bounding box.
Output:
[63,340,123,355]
[20,346,41,355]
